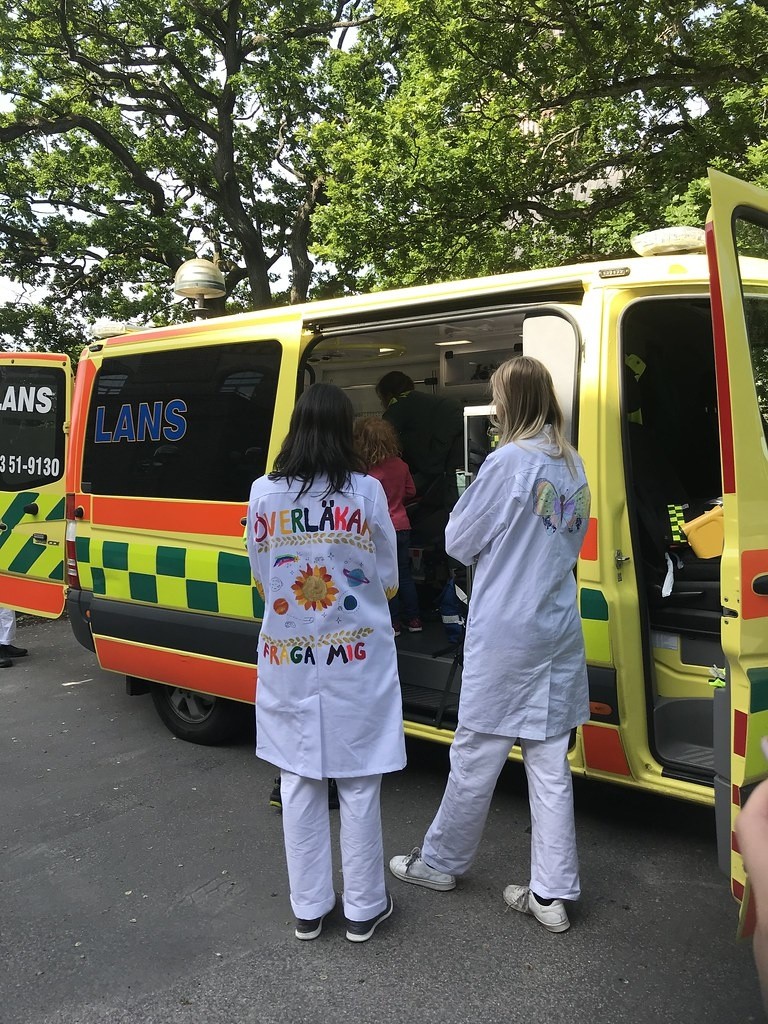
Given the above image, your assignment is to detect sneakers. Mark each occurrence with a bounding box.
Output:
[345,890,394,943]
[388,847,457,892]
[293,917,328,940]
[503,883,571,933]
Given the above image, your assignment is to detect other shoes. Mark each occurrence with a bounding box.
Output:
[268,777,282,810]
[0,644,27,657]
[391,624,401,637]
[408,617,423,632]
[0,658,13,668]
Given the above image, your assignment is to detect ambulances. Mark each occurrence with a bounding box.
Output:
[0,167,768,951]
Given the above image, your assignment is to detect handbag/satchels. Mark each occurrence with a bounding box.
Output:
[437,577,469,647]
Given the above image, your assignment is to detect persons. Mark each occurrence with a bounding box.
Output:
[388,358,592,934]
[735,776,768,1009]
[350,417,423,637]
[269,777,340,810]
[0,608,28,668]
[377,372,488,623]
[244,382,406,942]
